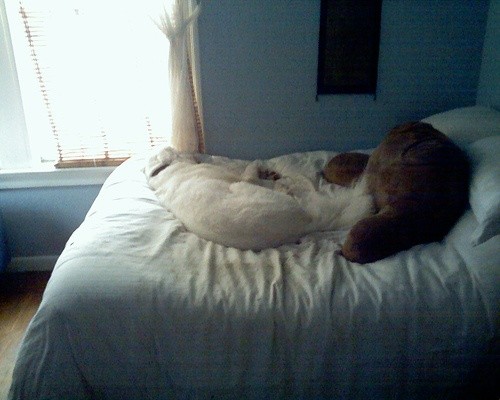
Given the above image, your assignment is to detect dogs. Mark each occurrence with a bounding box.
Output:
[143,142,373,251]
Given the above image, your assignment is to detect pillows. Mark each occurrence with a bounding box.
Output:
[465,135,499,247]
[417,106,499,154]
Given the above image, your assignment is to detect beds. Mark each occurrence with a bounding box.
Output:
[4,104,499,400]
